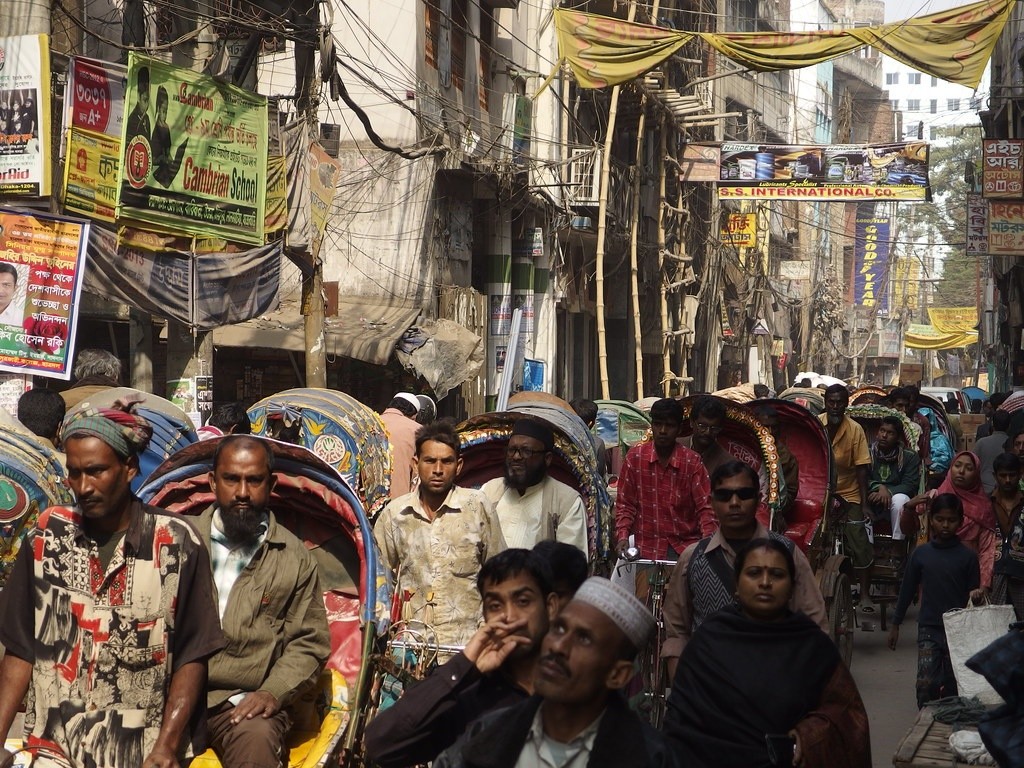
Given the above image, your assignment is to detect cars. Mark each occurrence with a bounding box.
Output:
[920,385,972,439]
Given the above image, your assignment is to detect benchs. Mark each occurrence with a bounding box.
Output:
[784,498,823,552]
[190,668,349,768]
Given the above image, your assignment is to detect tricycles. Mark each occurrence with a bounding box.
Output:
[0,386,956,768]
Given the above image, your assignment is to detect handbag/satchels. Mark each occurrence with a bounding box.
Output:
[942,594,1017,704]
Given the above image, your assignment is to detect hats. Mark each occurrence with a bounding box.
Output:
[394,392,421,413]
[576,574,659,647]
[510,422,553,451]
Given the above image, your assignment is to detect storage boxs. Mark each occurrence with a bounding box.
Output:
[953,414,985,454]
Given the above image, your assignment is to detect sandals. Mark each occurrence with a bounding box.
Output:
[859,596,876,614]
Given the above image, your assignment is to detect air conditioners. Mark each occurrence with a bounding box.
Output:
[572,149,604,202]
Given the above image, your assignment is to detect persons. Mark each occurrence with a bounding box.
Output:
[0,98,33,152]
[364,542,679,768]
[572,397,607,482]
[864,415,921,559]
[938,392,1011,440]
[374,392,458,503]
[889,493,984,709]
[754,384,769,400]
[0,408,224,768]
[19,346,121,449]
[617,398,873,768]
[173,435,332,768]
[777,379,857,396]
[125,66,173,175]
[375,422,507,718]
[865,383,954,483]
[479,420,588,564]
[212,401,254,438]
[0,262,24,327]
[899,410,1024,593]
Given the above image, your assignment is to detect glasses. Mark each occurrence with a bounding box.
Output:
[714,487,756,502]
[695,419,722,434]
[505,447,547,458]
